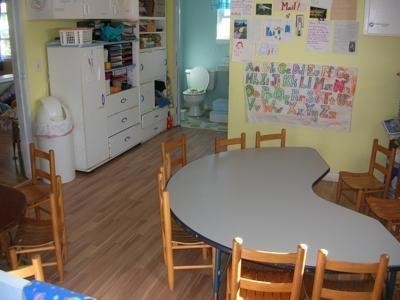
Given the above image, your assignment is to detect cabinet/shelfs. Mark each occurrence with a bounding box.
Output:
[114,1,135,22]
[105,84,143,160]
[25,1,114,21]
[100,37,137,92]
[134,0,169,144]
[45,41,109,173]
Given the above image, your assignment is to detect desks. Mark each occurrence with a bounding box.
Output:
[166,147,400,300]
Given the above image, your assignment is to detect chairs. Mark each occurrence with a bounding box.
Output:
[0,119,69,284]
[255,128,286,149]
[157,128,218,293]
[226,237,308,300]
[312,249,390,300]
[365,164,400,241]
[215,133,246,153]
[336,139,397,212]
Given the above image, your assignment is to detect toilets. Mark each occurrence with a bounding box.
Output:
[182,66,216,117]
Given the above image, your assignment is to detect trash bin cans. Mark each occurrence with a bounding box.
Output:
[33,97,76,184]
